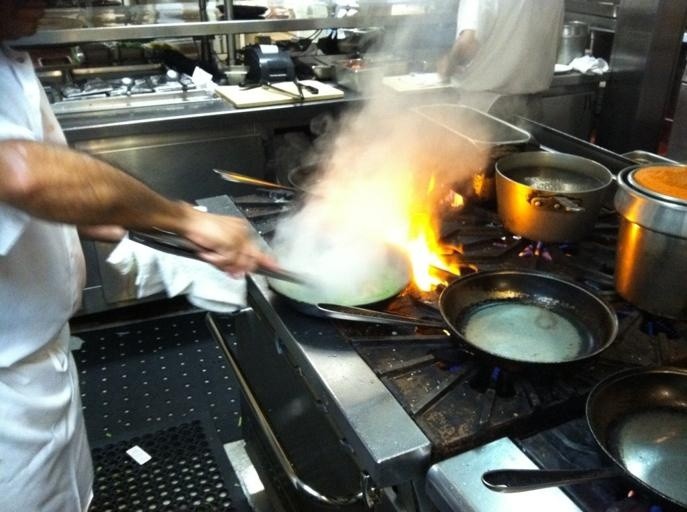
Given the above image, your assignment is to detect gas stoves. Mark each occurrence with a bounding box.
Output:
[193,189,686,488]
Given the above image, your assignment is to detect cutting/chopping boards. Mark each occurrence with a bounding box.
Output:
[382,69,456,95]
[214,77,345,108]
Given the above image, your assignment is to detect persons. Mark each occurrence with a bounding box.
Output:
[433,0,565,125]
[0,1,281,512]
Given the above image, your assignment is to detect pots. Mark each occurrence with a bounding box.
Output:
[556,19,588,67]
[481,366,687,512]
[258,226,413,311]
[493,151,619,248]
[211,159,334,207]
[612,161,685,324]
[314,268,622,370]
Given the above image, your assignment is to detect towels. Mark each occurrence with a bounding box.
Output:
[103,202,251,316]
[567,49,610,77]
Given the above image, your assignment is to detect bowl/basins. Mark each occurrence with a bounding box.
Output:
[276,38,312,53]
[215,4,270,16]
